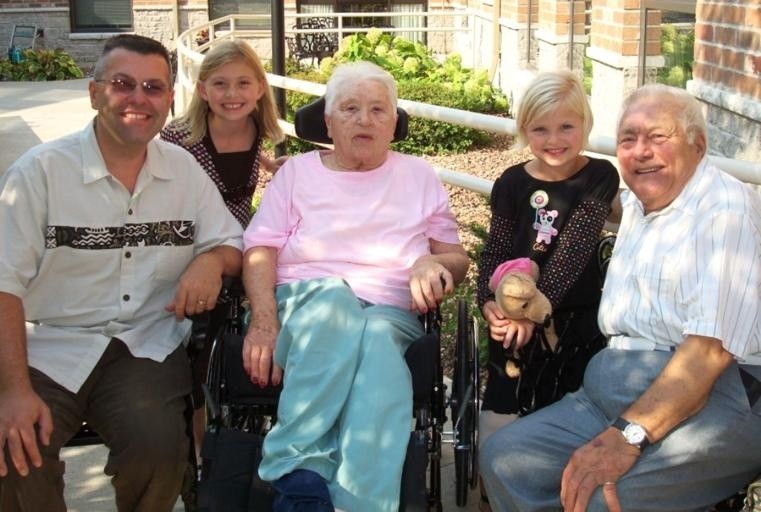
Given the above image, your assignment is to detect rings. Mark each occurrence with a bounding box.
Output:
[197,300,207,305]
[602,480,620,486]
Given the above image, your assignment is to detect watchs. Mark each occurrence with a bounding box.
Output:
[611,416,651,453]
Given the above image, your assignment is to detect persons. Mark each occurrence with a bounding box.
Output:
[240,58,472,512]
[482,82,761,512]
[0,33,245,512]
[157,39,290,229]
[476,67,619,511]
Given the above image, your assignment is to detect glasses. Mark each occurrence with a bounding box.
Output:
[97,79,171,96]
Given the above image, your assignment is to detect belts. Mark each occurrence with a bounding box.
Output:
[608,336,675,351]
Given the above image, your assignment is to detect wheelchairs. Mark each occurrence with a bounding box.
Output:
[173,94,482,512]
